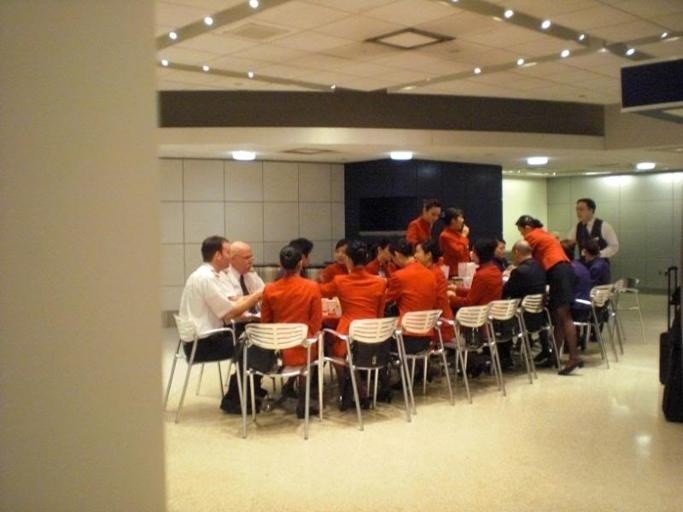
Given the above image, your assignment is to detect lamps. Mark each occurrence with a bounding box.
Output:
[391,152,410,158]
[637,161,656,168]
[234,153,256,160]
[527,157,548,166]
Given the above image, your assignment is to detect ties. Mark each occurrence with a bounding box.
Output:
[240,275,257,314]
[580,224,588,242]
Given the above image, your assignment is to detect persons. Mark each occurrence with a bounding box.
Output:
[567,199,618,341]
[497,240,548,370]
[407,200,440,260]
[438,207,468,280]
[260,246,322,420]
[271,238,313,281]
[318,239,351,285]
[583,238,613,341]
[411,240,455,381]
[385,238,436,389]
[177,236,267,416]
[322,240,385,413]
[364,239,396,279]
[447,239,503,378]
[226,241,265,324]
[492,236,509,272]
[559,238,592,353]
[515,216,584,375]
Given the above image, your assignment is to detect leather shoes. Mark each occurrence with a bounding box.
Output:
[221,374,267,414]
[591,334,597,341]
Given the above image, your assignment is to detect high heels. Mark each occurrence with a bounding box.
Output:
[337,395,352,411]
[357,397,370,407]
[296,406,313,417]
[378,392,392,402]
[558,359,583,374]
[455,354,484,377]
[414,372,431,383]
[282,384,296,398]
[542,355,558,367]
[501,359,514,370]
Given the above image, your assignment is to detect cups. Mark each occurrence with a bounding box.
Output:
[326,300,337,315]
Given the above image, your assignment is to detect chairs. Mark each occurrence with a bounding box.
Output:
[613,279,628,355]
[518,295,561,377]
[438,305,506,403]
[389,309,454,408]
[569,283,619,373]
[322,314,411,427]
[234,322,320,442]
[164,312,244,422]
[617,277,647,344]
[483,298,533,385]
